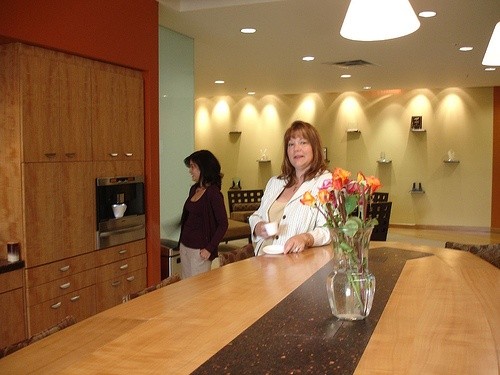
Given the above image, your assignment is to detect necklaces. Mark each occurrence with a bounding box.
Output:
[293,187,297,192]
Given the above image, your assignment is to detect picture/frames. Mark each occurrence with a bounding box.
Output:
[411,116,422,129]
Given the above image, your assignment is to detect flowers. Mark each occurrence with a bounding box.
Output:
[298,169,382,313]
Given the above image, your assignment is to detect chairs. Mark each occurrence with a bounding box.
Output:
[228,190,263,213]
[352,191,392,241]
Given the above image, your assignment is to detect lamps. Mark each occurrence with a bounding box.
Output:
[340,0,420,42]
[482,20,500,66]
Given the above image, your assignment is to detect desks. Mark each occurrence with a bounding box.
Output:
[0,241,500,375]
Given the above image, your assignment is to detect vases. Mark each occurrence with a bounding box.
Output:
[327,226,377,320]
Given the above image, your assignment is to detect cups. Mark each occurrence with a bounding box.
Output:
[265,222,278,236]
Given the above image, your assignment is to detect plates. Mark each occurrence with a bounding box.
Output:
[262,245,284,254]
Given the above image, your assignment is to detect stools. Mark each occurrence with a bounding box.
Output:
[218,203,261,246]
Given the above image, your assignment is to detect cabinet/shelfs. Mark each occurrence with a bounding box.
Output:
[0,41,148,359]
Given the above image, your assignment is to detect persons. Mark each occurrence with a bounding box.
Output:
[250,120,339,256]
[176,150,228,278]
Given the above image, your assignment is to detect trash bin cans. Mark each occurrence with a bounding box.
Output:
[160,239,182,280]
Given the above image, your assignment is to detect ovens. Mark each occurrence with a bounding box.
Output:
[95,175,146,250]
